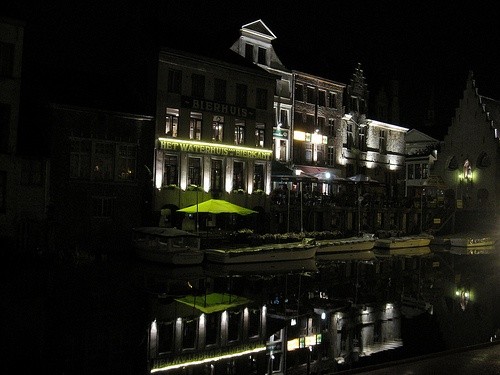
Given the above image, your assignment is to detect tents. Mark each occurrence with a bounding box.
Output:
[175,198,262,240]
[174,274,256,314]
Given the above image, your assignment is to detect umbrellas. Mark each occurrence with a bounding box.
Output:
[273,167,319,196]
[306,172,346,196]
[343,172,386,201]
[267,280,381,320]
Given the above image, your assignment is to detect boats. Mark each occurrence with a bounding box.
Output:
[206,238,321,264]
[450,233,497,246]
[375,232,435,249]
[316,234,379,253]
[374,246,431,258]
[315,250,378,263]
[204,258,320,279]
[449,246,497,255]
[133,226,204,265]
